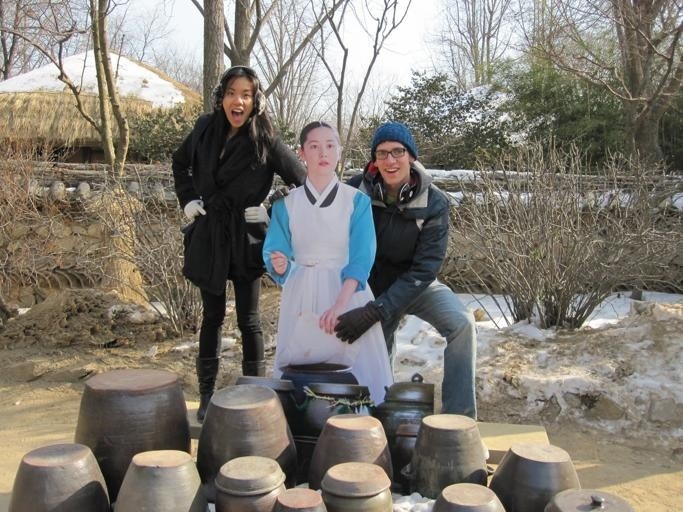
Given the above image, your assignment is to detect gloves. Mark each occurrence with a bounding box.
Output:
[243,203,270,228]
[183,200,206,221]
[333,300,381,344]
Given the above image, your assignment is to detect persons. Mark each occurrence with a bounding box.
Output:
[167,65,307,421]
[262,120,393,407]
[268,121,479,422]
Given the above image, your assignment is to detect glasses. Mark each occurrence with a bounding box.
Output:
[372,147,408,159]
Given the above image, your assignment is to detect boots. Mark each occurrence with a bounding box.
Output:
[241,359,266,377]
[192,358,220,423]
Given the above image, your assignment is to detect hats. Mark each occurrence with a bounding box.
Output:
[370,122,418,164]
